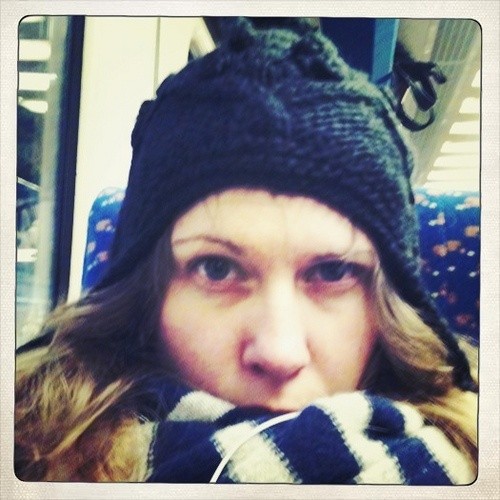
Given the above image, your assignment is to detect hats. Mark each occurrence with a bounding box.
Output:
[113,17,474,392]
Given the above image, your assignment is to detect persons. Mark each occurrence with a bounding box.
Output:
[14,17,480,486]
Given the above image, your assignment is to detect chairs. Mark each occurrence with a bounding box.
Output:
[75,175,480,372]
